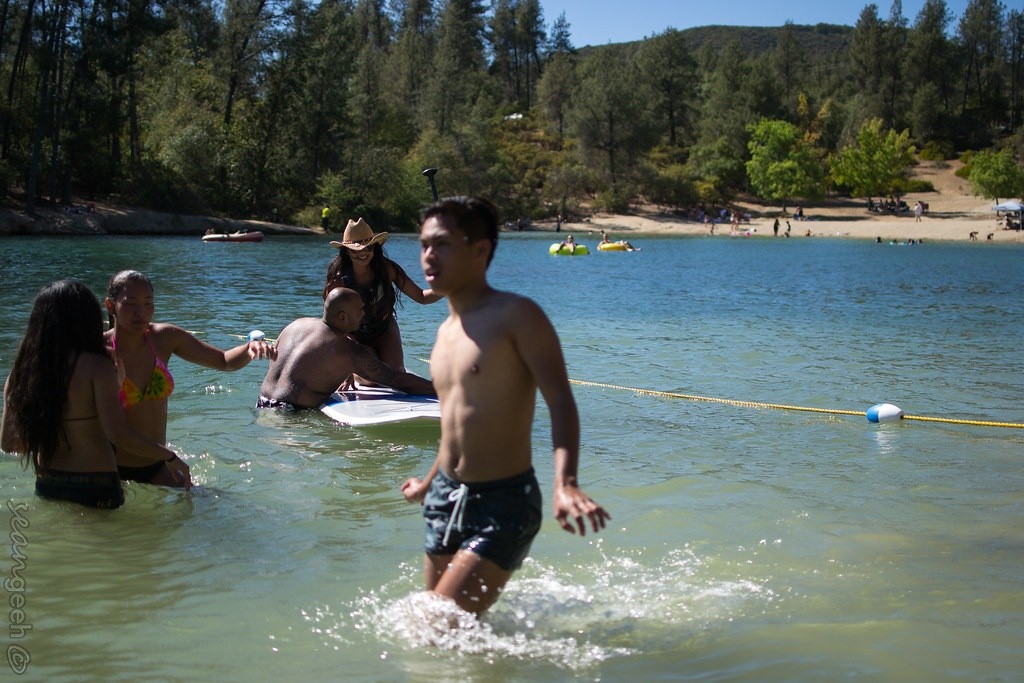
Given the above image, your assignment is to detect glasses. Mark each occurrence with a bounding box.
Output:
[351,245,374,253]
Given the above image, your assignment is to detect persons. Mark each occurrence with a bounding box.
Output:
[102,268,277,487]
[556,235,577,254]
[710,223,715,234]
[588,229,642,254]
[515,212,567,232]
[322,205,331,231]
[773,196,1024,245]
[0,280,125,510]
[323,218,446,390]
[705,213,708,228]
[399,197,612,618]
[256,285,437,412]
[729,212,739,231]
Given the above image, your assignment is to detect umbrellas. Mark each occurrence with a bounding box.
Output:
[991,201,1024,211]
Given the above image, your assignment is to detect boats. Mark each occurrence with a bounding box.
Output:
[202,231,264,243]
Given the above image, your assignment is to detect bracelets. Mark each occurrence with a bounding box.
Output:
[166,453,176,462]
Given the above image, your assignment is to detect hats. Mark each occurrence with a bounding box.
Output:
[329,217,388,251]
